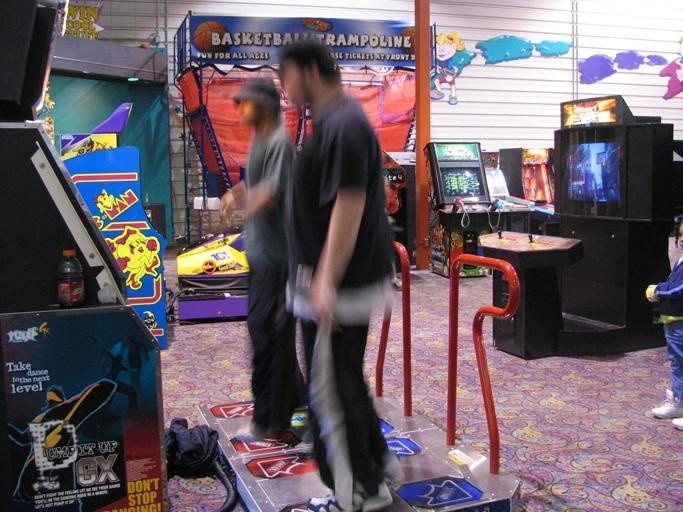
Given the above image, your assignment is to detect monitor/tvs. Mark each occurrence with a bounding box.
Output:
[562,141,620,203]
[440,167,484,196]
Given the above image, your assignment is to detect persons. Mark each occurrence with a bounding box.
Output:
[216,77,307,442]
[270,40,398,512]
[645,217,683,430]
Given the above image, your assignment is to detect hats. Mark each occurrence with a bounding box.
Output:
[230,77,284,117]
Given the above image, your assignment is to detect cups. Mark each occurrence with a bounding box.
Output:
[193,196,220,210]
[98,285,116,306]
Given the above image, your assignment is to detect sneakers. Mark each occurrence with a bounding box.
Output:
[650,389,683,419]
[307,481,395,512]
[671,417,683,432]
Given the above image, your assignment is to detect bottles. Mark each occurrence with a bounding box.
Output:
[56,249,85,307]
